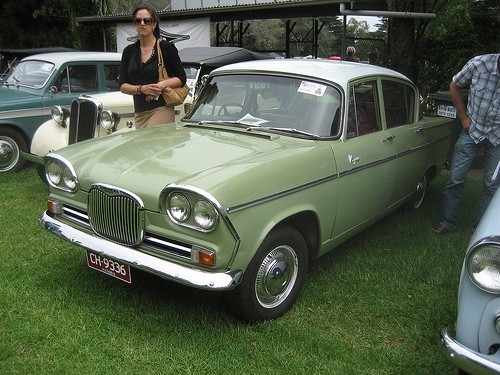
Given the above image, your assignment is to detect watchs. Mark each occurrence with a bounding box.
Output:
[136,85,142,95]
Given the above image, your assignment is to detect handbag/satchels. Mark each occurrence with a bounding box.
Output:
[156,38,190,106]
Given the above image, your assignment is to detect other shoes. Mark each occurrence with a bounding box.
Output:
[431,223,449,234]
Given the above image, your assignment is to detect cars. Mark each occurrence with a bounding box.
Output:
[40,58,457,321]
[0,47,275,186]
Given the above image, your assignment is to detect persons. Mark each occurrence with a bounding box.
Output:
[118,5,188,129]
[430,53,500,233]
[344,46,360,62]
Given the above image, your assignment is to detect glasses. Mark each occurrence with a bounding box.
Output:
[133,18,155,25]
